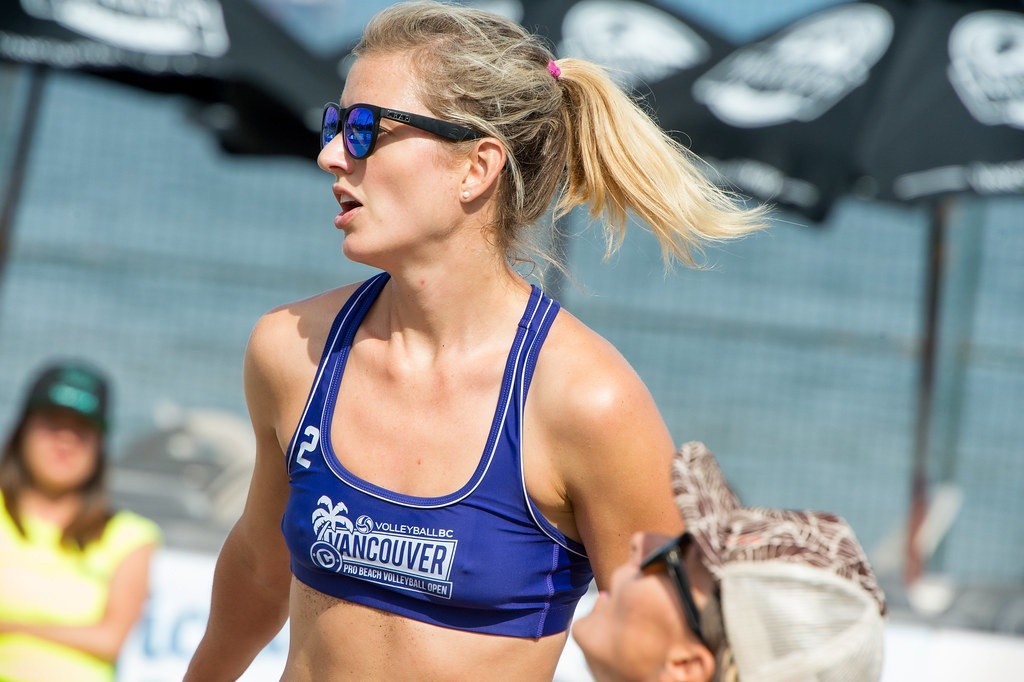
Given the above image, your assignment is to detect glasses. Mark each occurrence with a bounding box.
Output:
[639,532,715,655]
[319,101,490,161]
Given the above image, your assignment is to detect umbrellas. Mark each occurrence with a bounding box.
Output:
[0,0,1024,585]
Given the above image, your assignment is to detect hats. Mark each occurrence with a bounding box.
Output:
[671,441,888,682]
[32,363,106,423]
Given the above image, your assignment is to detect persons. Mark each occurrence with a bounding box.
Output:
[184,4,771,682]
[571,442,887,682]
[0,360,159,682]
